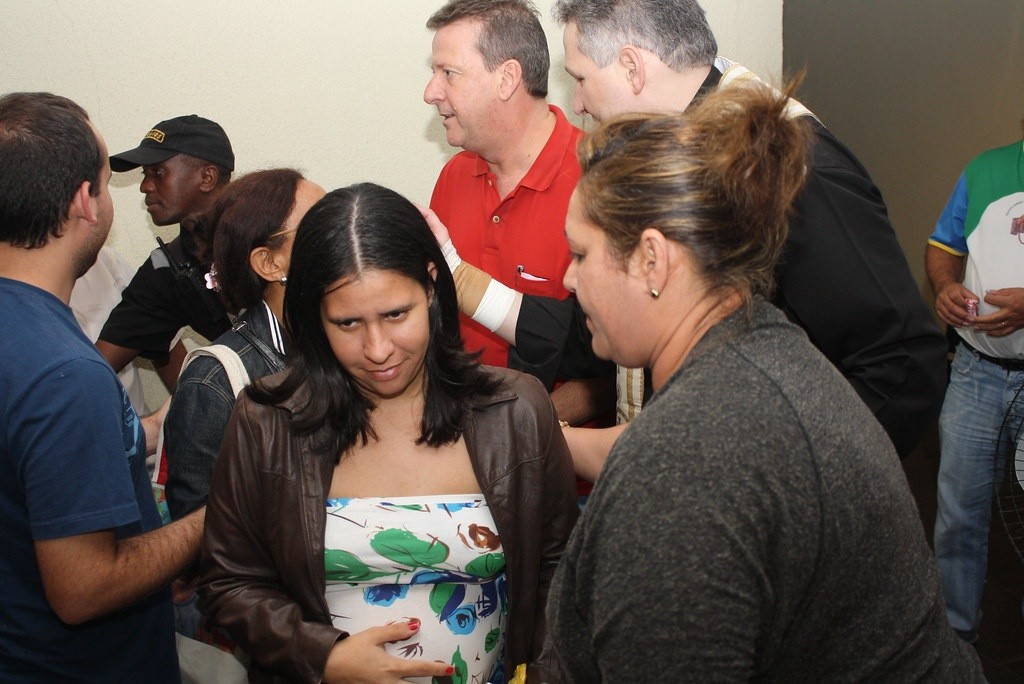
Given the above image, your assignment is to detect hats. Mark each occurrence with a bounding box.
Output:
[108,114,235,172]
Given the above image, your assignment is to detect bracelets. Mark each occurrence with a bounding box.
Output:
[559,420,569,427]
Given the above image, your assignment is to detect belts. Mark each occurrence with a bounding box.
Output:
[961,337,1024,371]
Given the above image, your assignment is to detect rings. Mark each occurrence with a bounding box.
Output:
[1002,321,1007,328]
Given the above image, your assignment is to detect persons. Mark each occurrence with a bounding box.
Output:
[197,182,580,684]
[922,138,1024,641]
[70,246,147,418]
[0,93,208,684]
[422,0,616,503]
[168,168,327,521]
[94,113,234,373]
[538,92,987,683]
[552,0,948,459]
